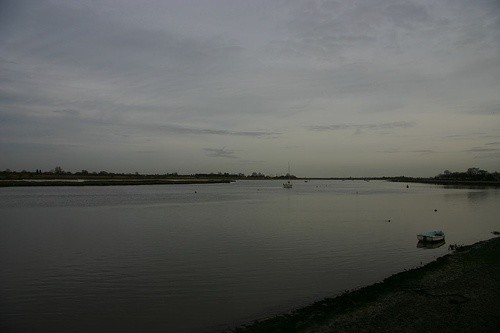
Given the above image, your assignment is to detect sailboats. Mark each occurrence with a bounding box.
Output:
[283,167,293,189]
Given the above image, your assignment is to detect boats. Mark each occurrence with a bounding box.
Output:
[417,229,445,242]
[417,241,446,248]
